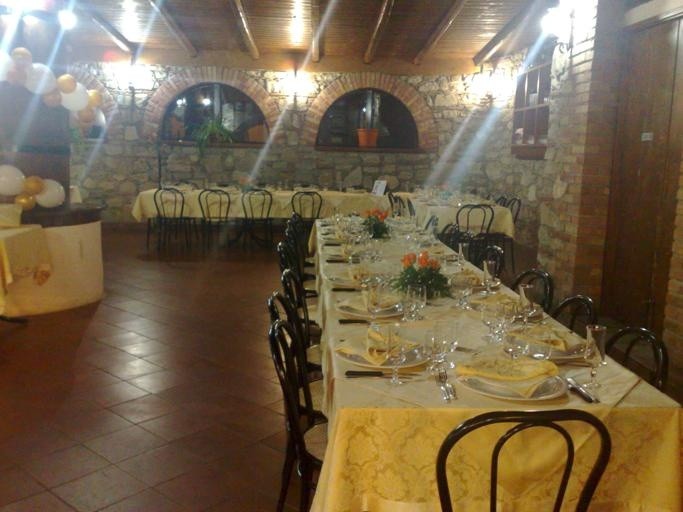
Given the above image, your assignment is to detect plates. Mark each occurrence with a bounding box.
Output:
[456,378,567,401]
[448,277,501,290]
[335,350,429,369]
[540,327,594,366]
[334,294,404,318]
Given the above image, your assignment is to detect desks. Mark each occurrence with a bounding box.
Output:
[0,224,53,325]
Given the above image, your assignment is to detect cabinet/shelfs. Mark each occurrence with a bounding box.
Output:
[511,60,551,161]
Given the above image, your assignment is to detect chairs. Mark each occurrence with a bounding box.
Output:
[278,241,317,299]
[601,326,668,390]
[421,216,438,235]
[388,192,394,208]
[154,187,189,255]
[476,245,504,278]
[291,191,322,219]
[241,189,272,252]
[456,231,474,259]
[267,289,324,462]
[407,199,415,216]
[494,196,507,208]
[268,319,327,512]
[466,236,499,267]
[552,295,599,344]
[287,220,316,274]
[283,228,315,287]
[506,198,521,225]
[456,205,494,236]
[509,266,552,314]
[436,409,611,512]
[282,269,321,361]
[394,197,405,209]
[482,193,494,201]
[198,189,230,252]
[439,224,458,247]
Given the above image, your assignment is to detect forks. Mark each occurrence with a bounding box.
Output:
[438,369,457,400]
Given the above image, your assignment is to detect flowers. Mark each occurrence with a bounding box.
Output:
[386,251,450,298]
[362,208,389,238]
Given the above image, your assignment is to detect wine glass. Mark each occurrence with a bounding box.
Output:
[529,331,551,360]
[433,320,459,369]
[456,242,469,272]
[480,299,524,347]
[405,284,425,321]
[424,331,449,375]
[451,277,473,311]
[344,238,356,268]
[480,260,496,295]
[509,336,527,361]
[386,324,407,386]
[360,276,385,326]
[583,324,606,388]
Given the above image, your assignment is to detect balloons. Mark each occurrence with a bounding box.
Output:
[36,178,66,208]
[0,164,25,197]
[0,47,107,128]
[15,194,37,212]
[23,174,45,196]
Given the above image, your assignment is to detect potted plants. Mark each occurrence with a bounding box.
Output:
[194,114,236,150]
[354,116,379,147]
